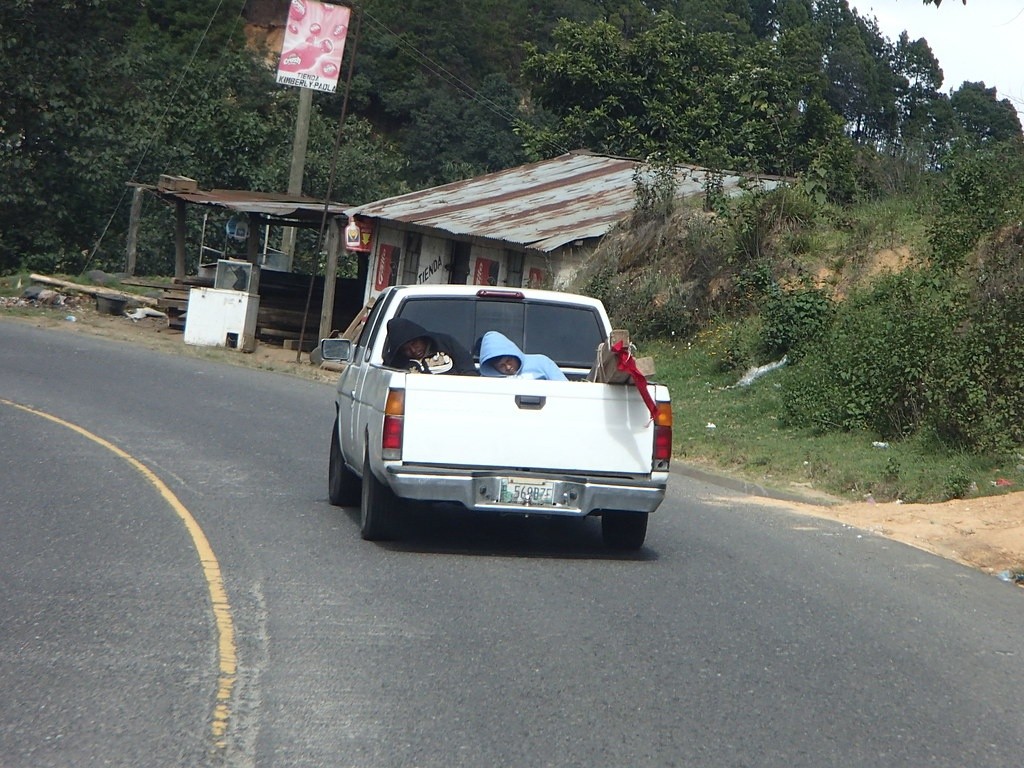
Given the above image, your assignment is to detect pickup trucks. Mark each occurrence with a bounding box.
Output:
[319,284,674,549]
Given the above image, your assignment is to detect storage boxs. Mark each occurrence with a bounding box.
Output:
[158,174,197,192]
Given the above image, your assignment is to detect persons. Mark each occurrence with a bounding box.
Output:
[383,318,480,377]
[479,331,569,381]
